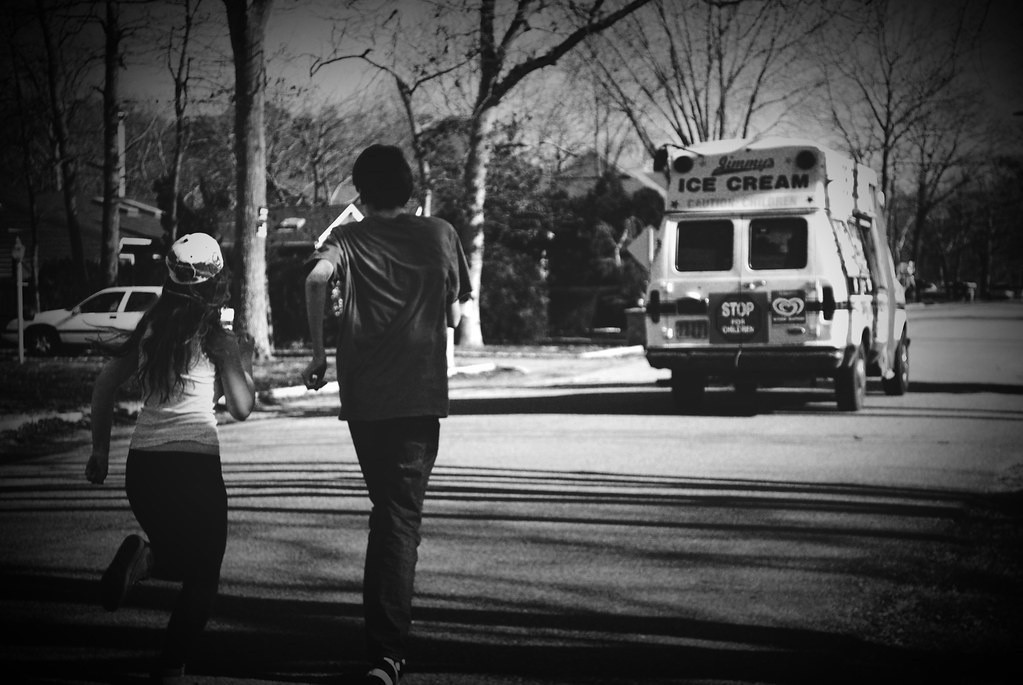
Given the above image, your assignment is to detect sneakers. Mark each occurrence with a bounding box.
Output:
[365,656,403,685]
[96,536,149,610]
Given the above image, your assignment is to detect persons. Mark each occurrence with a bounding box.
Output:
[83,232,259,676]
[299,142,478,683]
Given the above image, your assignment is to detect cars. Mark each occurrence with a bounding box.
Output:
[4,283,255,356]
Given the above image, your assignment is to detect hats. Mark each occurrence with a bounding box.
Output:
[165,233,222,285]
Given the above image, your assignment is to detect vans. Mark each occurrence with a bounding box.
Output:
[643,138,914,407]
